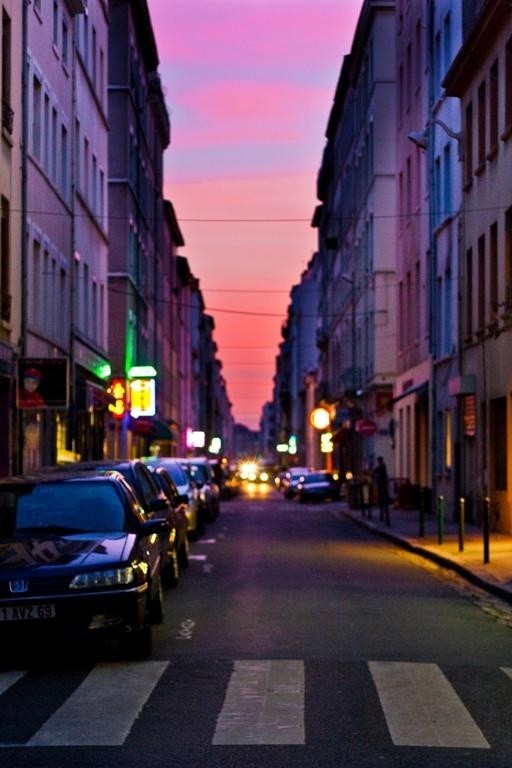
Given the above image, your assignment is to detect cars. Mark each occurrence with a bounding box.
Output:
[138,458,207,542]
[182,460,220,520]
[60,461,193,588]
[209,460,237,498]
[272,468,333,504]
[2,470,172,642]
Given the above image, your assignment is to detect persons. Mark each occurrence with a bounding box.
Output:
[375,455,393,507]
[18,367,45,405]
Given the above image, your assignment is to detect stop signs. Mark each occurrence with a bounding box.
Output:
[356,419,377,438]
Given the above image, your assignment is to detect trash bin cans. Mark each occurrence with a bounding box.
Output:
[347,479,371,510]
